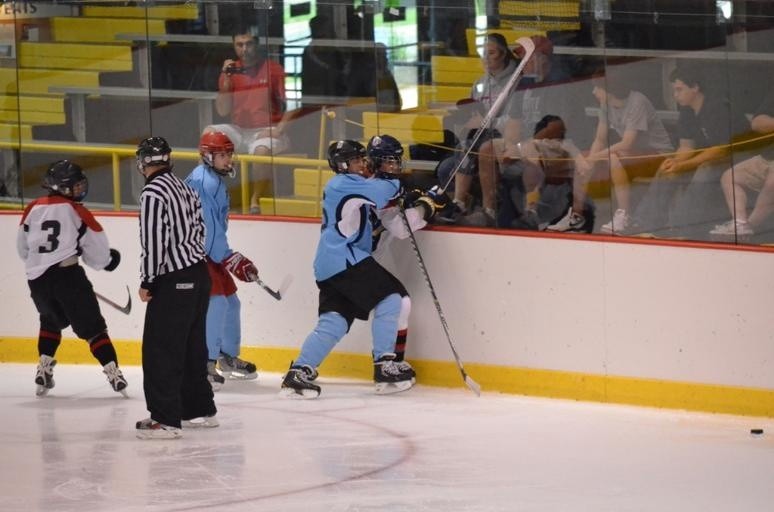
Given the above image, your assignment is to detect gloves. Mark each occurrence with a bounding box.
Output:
[105,249,120,271]
[415,185,447,220]
[402,189,425,208]
[222,252,259,281]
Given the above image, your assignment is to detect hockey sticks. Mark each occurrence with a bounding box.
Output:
[248,271,281,301]
[95,284,131,315]
[397,196,481,397]
[440,36,534,196]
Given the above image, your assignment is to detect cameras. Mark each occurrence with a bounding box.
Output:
[223,63,250,75]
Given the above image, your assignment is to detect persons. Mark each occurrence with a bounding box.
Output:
[279,133,449,396]
[133,134,220,433]
[16,159,131,393]
[204,11,774,239]
[364,133,443,379]
[184,133,261,386]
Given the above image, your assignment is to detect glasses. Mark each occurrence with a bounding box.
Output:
[199,132,236,178]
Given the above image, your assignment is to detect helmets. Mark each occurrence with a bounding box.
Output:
[42,160,88,202]
[328,140,366,175]
[367,135,404,179]
[136,137,172,176]
